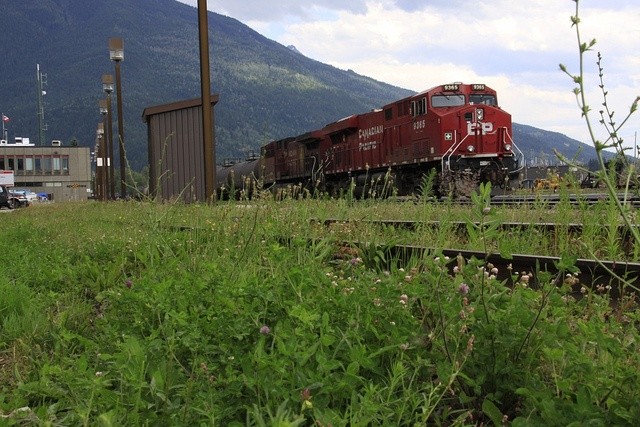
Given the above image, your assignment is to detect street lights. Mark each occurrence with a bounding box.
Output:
[97,121,104,201]
[99,98,109,200]
[101,72,115,198]
[108,37,127,198]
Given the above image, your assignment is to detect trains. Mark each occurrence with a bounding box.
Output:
[216,81,525,201]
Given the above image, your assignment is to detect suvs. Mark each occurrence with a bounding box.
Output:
[0,184,37,209]
